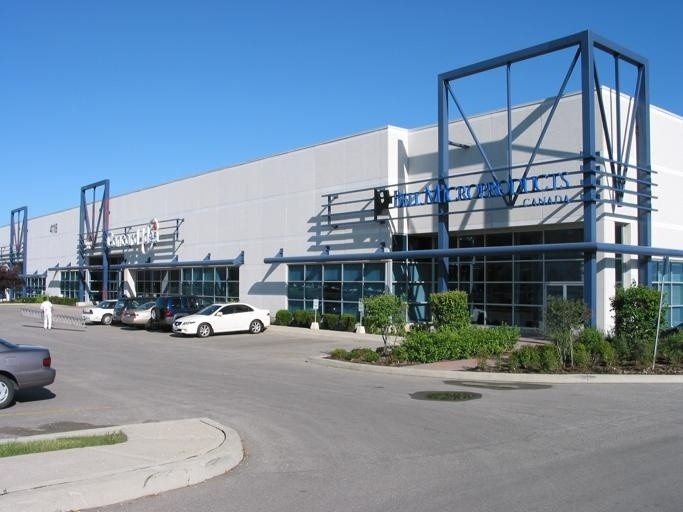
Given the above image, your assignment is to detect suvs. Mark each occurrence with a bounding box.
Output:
[151,295,215,331]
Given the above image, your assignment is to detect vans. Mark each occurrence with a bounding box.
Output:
[112,298,156,322]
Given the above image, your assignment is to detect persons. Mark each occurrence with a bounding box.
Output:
[40,296,54,331]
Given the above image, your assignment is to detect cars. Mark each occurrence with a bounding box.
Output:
[0,337,55,409]
[120,301,157,326]
[172,302,271,337]
[83,300,118,325]
